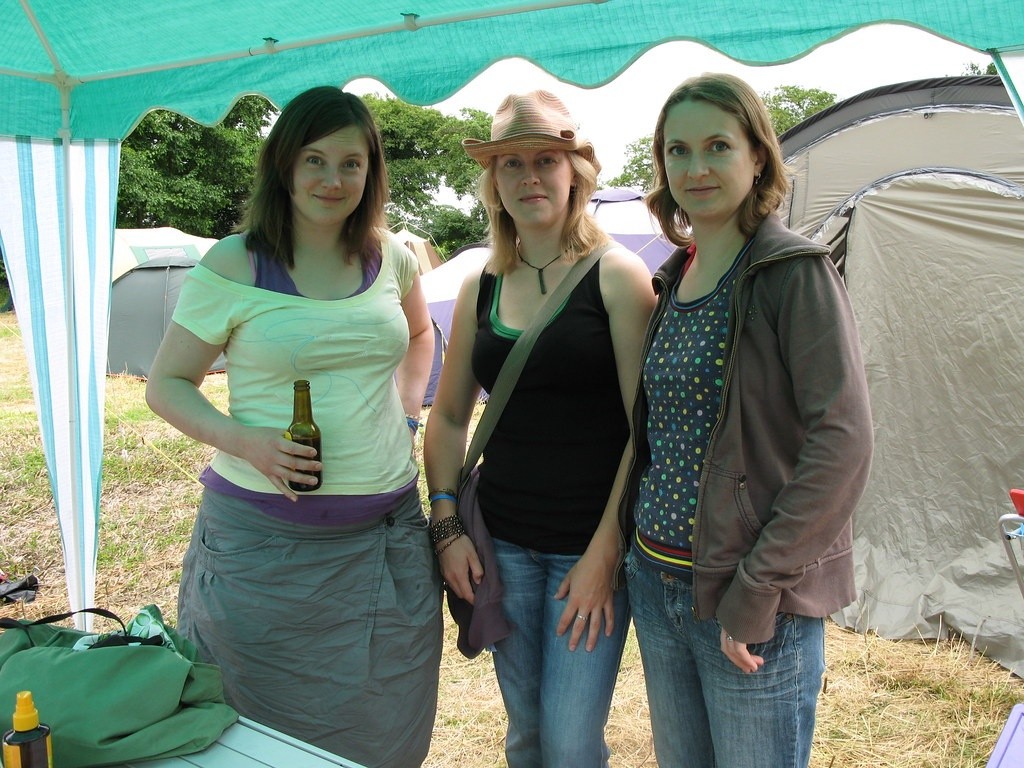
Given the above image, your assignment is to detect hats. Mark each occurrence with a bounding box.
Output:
[460,90,602,177]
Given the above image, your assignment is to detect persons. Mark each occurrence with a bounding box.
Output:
[612,73,875,768]
[424,88,659,768]
[144,85,445,768]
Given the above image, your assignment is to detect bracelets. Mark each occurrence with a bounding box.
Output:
[404,414,423,434]
[428,487,465,557]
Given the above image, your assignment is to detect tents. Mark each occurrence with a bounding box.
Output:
[106,187,692,407]
[776,77,1024,681]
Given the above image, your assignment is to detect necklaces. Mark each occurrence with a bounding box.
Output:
[517,241,572,295]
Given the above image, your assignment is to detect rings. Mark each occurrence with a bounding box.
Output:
[726,633,734,641]
[577,614,587,621]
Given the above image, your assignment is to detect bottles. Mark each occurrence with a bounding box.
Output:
[286,379,321,492]
